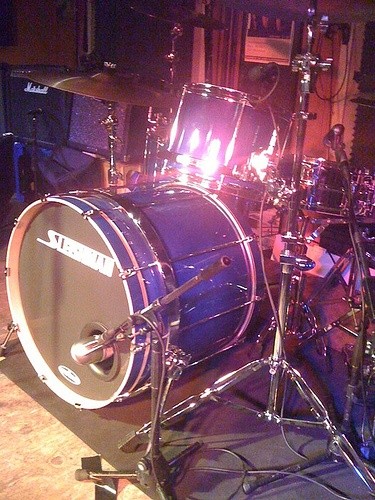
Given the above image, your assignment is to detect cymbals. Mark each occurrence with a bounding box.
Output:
[22,60,182,109]
[234,0,375,25]
[125,1,231,32]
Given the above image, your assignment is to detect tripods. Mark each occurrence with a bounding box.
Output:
[75,53,375,499]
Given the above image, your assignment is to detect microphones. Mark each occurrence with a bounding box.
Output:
[26,107,42,115]
[249,62,276,83]
[69,332,115,364]
[322,123,345,148]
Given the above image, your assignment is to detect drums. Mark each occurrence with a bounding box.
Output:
[5,190,266,412]
[163,80,306,177]
[300,158,352,213]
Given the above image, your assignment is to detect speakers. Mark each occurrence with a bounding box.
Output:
[5,64,69,150]
[62,92,147,164]
[39,146,102,200]
